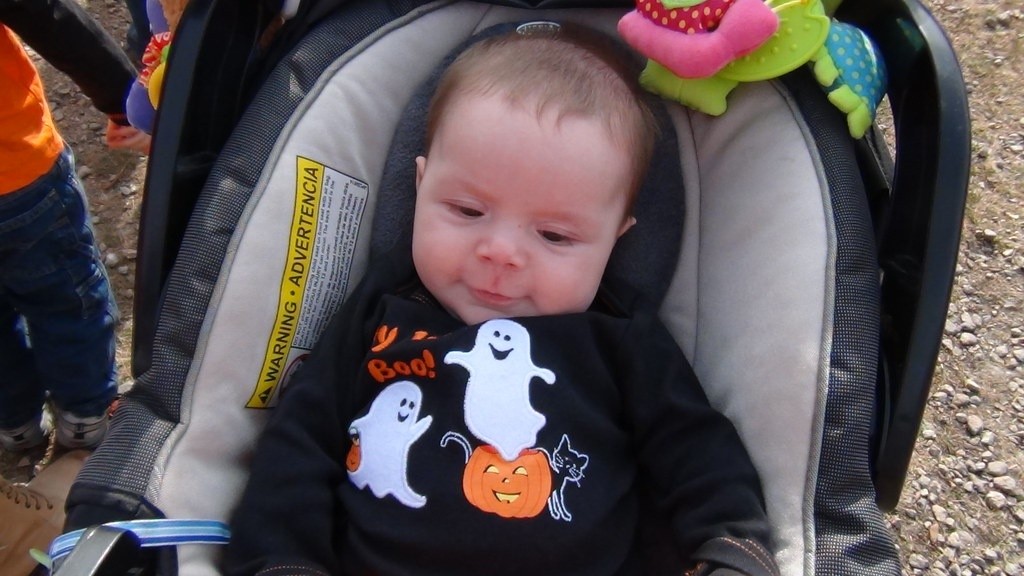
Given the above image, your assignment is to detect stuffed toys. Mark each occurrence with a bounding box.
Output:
[124,0,191,137]
[614,0,887,140]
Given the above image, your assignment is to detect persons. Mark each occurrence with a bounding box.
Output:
[0,450,94,576]
[105,0,155,156]
[219,12,783,576]
[0,0,157,464]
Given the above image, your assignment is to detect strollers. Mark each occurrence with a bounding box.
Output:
[24,0,969,576]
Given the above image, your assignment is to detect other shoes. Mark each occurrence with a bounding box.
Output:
[0,450,92,576]
[54,401,110,449]
[0,397,55,451]
